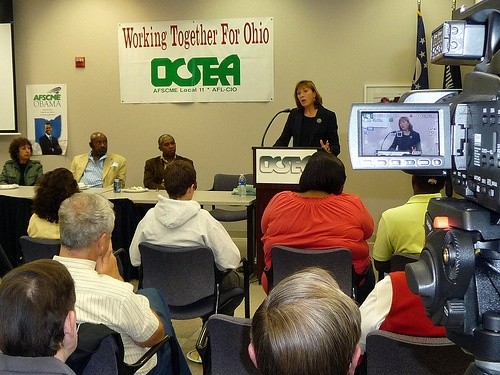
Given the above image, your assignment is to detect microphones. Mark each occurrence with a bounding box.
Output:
[261,109,290,146]
[381,131,396,150]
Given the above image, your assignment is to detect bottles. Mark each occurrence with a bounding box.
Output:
[239,174,247,198]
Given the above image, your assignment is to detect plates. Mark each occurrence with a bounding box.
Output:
[120,188,148,193]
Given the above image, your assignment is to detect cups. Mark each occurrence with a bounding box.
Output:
[114,179,121,193]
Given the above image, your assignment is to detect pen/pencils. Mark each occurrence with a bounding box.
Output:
[114,248,124,256]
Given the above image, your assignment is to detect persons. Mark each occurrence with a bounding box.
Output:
[38,122,62,155]
[271,80,340,157]
[248,267,362,374]
[0,137,43,186]
[260,151,375,304]
[372,167,446,272]
[70,131,127,189]
[26,167,80,237]
[129,159,244,329]
[0,259,78,375]
[358,271,450,365]
[144,134,194,190]
[53,193,171,375]
[388,116,422,156]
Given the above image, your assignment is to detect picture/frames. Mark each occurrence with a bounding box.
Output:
[363,85,411,104]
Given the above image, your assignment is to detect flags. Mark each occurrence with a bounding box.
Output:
[443,7,463,94]
[411,10,429,91]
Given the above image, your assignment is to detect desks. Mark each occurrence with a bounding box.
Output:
[0,185,113,277]
[103,189,257,263]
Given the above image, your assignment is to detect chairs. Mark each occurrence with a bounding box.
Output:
[366,330,476,375]
[138,243,243,319]
[264,246,362,301]
[65,323,180,375]
[196,313,257,375]
[135,288,192,375]
[209,174,255,221]
[111,199,140,281]
[18,236,61,265]
